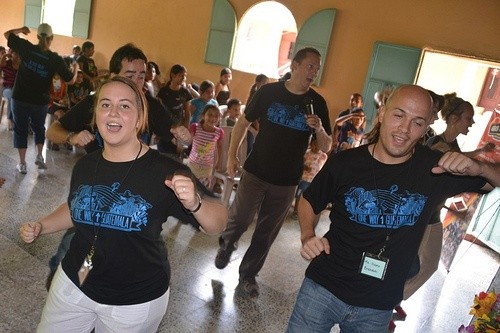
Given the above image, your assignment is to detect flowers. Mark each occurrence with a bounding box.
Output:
[457,286,500,333]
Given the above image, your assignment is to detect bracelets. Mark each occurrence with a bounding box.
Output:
[37,222,43,234]
[66,132,76,147]
[182,192,201,214]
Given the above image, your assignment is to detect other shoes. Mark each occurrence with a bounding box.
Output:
[64,142,73,151]
[9,128,14,132]
[239,274,260,295]
[215,247,233,269]
[47,141,59,151]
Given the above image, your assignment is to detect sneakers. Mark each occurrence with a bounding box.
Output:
[35,155,47,169]
[16,162,27,174]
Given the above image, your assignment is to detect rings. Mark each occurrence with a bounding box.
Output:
[183,186,186,192]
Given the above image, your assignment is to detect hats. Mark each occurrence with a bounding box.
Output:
[37,23,52,38]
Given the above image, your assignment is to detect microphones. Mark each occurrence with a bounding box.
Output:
[303,97,316,134]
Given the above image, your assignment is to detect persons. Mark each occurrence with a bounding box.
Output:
[285,83,500,332]
[44,42,194,329]
[0,23,268,206]
[20,77,228,333]
[216,48,332,297]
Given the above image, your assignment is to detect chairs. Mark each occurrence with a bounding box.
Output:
[214,126,240,202]
